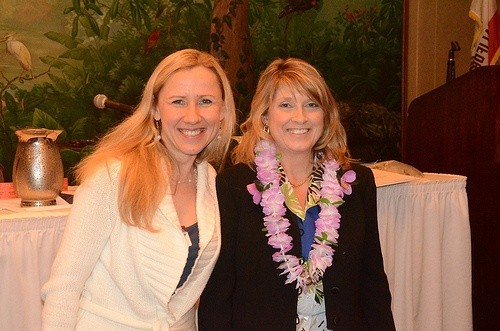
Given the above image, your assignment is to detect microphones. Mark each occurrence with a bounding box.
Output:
[93,94,137,115]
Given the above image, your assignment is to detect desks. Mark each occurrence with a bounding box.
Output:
[0,164,471,331]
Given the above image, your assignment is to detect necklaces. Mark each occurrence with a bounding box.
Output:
[286,171,314,188]
[168,167,199,185]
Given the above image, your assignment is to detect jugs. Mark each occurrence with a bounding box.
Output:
[14,128,64,208]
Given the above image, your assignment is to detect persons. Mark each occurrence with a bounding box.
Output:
[40,48,237,331]
[196,56,395,331]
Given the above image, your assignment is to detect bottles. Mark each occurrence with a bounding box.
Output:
[446,41,459,83]
